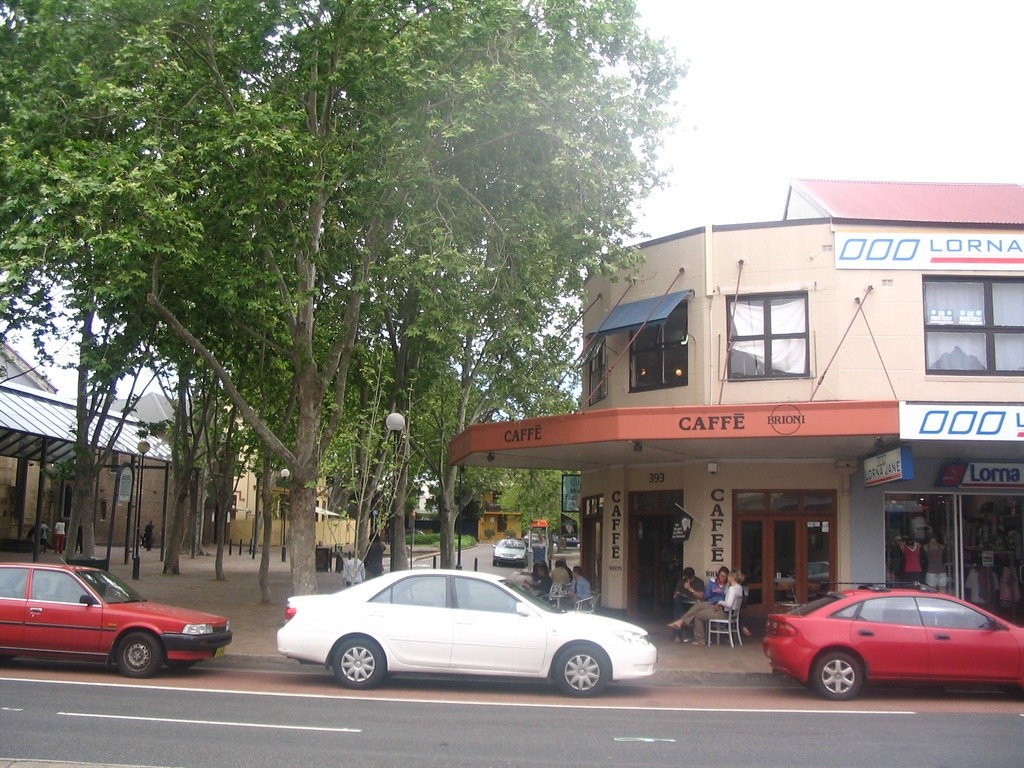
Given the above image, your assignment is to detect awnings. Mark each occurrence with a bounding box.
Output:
[586,288,695,340]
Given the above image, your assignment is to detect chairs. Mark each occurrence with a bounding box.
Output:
[708,595,743,648]
[574,595,599,614]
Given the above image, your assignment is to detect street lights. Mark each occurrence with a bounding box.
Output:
[132,441,151,580]
[281,469,290,562]
[386,413,405,573]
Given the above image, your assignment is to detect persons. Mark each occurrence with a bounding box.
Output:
[363,534,386,581]
[704,566,752,637]
[672,567,705,643]
[27,520,51,553]
[54,517,65,554]
[666,568,744,645]
[523,558,591,612]
[342,549,366,587]
[144,520,154,552]
[899,537,922,582]
[75,520,84,554]
[923,539,948,591]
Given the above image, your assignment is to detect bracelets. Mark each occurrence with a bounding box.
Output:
[690,589,694,594]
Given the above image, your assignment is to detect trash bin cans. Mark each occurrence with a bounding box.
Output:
[66,555,108,599]
[316,548,331,572]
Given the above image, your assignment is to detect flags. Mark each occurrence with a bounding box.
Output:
[671,505,694,544]
[560,513,577,540]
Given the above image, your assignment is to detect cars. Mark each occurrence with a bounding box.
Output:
[763,584,1024,701]
[0,562,233,679]
[277,569,658,699]
[492,538,529,568]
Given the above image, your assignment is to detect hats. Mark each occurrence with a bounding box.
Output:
[683,568,695,579]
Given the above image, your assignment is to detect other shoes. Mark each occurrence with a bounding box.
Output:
[742,628,752,636]
[691,640,706,645]
[667,624,681,628]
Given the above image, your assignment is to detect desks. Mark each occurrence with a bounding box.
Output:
[545,594,568,608]
[685,600,715,643]
